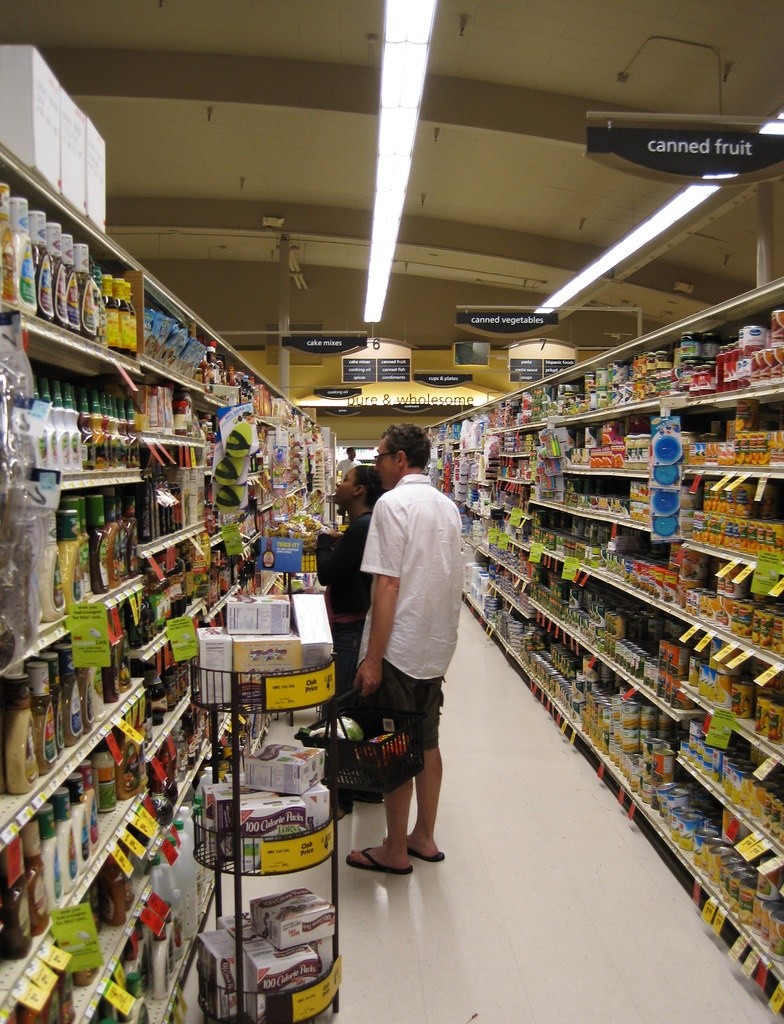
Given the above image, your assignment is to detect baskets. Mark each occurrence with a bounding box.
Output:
[294,675,426,792]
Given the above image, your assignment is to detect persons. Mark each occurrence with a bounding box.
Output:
[347,422,463,875]
[316,465,390,822]
[336,447,363,515]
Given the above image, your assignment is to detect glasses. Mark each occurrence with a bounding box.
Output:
[374,452,392,465]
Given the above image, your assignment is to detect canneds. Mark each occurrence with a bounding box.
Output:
[484,310,784,964]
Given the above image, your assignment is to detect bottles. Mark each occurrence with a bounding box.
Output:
[0,182,192,1024]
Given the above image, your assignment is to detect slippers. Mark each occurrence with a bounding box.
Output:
[384,833,445,862]
[346,848,414,875]
